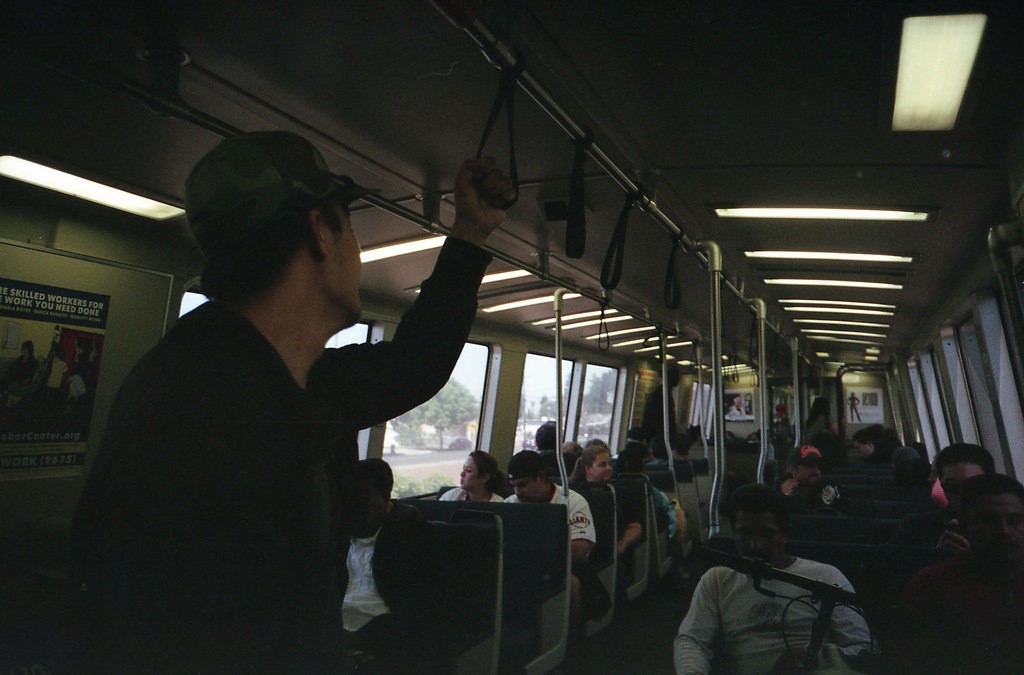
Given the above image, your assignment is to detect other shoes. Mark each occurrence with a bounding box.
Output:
[672,572,690,592]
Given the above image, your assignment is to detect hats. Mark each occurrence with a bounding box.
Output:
[789,445,823,466]
[507,450,547,479]
[892,446,920,466]
[184,130,382,263]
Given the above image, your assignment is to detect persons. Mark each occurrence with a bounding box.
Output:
[70,129,519,675]
[0,336,98,432]
[673,482,881,675]
[324,354,997,674]
[724,391,753,417]
[894,473,1024,675]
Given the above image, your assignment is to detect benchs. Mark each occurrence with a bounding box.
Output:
[421,509,504,675]
[613,436,760,580]
[779,512,900,542]
[702,536,940,643]
[412,475,618,674]
[545,476,650,601]
